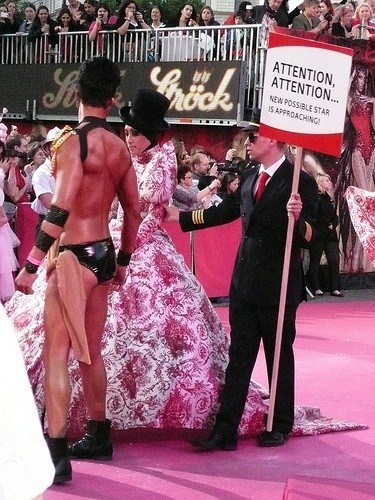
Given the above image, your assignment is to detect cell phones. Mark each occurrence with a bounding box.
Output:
[99,14,103,18]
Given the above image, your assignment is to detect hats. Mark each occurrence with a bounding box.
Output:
[43,126,61,154]
[118,89,171,131]
[239,106,262,132]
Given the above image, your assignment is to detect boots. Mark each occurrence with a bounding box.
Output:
[64,418,115,460]
[42,432,74,484]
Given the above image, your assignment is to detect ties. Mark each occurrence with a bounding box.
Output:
[253,172,270,203]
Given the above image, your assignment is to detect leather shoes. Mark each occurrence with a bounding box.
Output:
[191,433,237,451]
[253,431,291,447]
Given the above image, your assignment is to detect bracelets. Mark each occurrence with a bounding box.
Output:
[27,256,41,266]
[25,261,39,273]
[207,186,212,192]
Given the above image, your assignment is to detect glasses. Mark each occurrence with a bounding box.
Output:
[185,176,192,179]
[34,151,47,157]
[247,131,257,144]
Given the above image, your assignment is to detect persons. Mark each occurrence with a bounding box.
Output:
[0,0,375,500]
[346,64,375,193]
[162,108,327,450]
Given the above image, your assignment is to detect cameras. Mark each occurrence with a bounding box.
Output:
[132,10,138,15]
[324,13,332,21]
[210,162,238,173]
[2,149,27,161]
